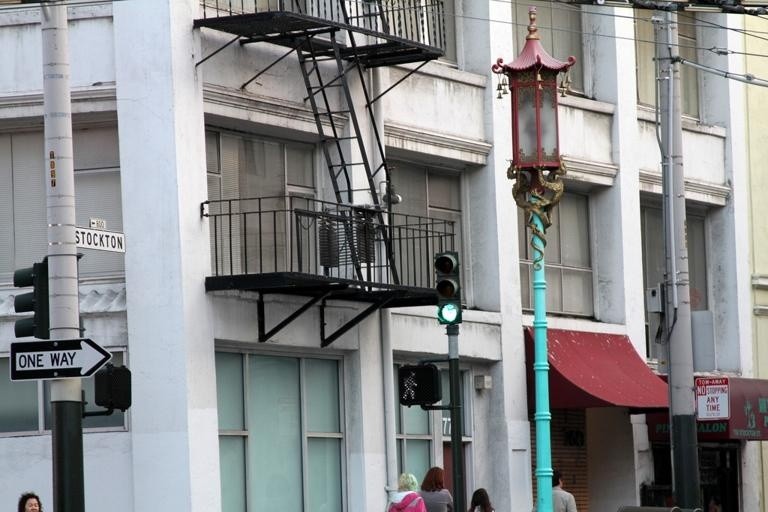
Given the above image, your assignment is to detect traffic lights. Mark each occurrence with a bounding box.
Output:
[13,254,50,339]
[432,253,463,326]
[399,365,442,404]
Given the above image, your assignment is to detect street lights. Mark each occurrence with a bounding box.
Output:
[493,6,577,510]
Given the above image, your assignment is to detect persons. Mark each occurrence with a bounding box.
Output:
[420,467,454,512]
[386,473,428,512]
[552,468,578,512]
[468,488,496,512]
[17,491,42,511]
